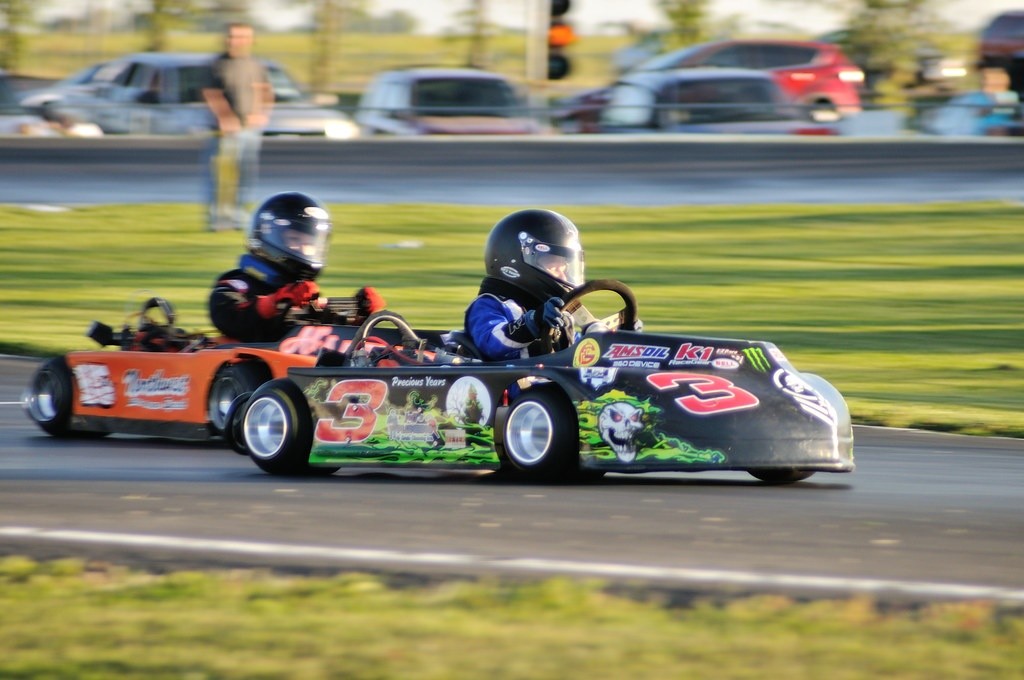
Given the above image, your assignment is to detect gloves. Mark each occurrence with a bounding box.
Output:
[355,286,387,317]
[634,318,643,332]
[524,297,564,339]
[255,280,319,321]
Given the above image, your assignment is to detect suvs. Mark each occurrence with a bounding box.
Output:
[550,40,862,131]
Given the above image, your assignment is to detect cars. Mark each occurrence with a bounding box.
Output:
[1,74,104,138]
[25,54,359,145]
[350,66,553,137]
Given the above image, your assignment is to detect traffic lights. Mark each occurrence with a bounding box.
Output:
[546,0,577,78]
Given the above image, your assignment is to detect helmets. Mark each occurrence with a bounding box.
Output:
[484,209,585,308]
[247,192,330,279]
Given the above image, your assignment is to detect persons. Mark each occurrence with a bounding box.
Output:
[204,24,272,233]
[207,192,384,345]
[465,209,644,360]
